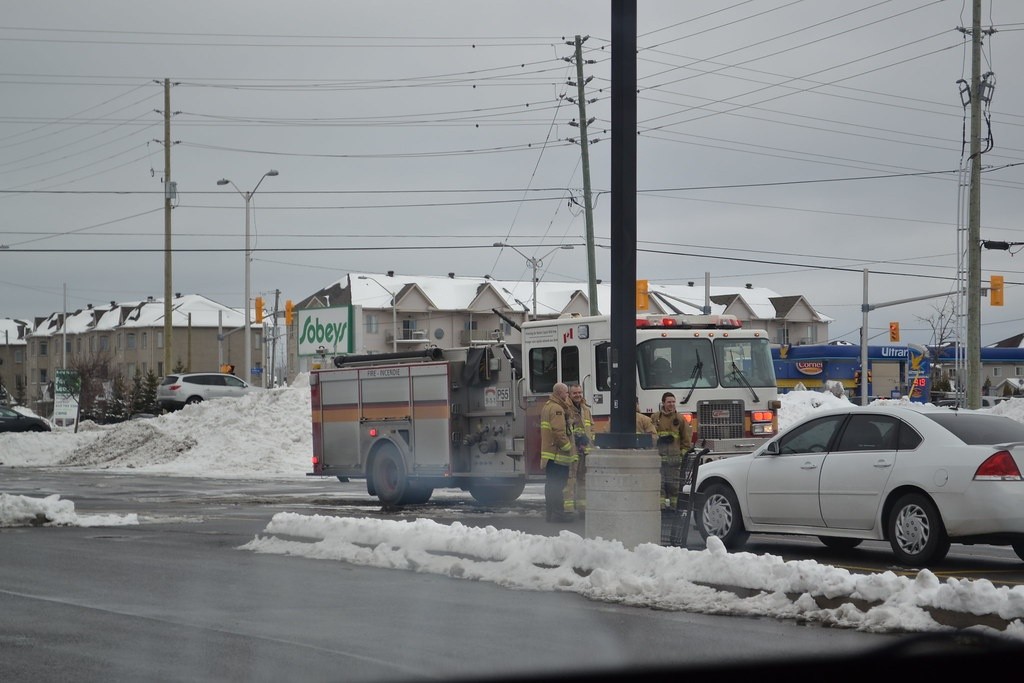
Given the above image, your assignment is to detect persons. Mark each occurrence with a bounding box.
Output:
[650,392,690,514]
[540,382,577,523]
[563,383,595,516]
[603,396,659,448]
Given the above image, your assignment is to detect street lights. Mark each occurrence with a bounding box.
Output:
[216,169,279,384]
[493,243,574,319]
[357,276,397,353]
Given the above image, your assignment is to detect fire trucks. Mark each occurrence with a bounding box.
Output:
[305,314,781,508]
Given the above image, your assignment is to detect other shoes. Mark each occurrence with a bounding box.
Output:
[670,496,678,511]
[576,500,585,511]
[563,500,574,512]
[546,505,575,523]
[660,498,666,511]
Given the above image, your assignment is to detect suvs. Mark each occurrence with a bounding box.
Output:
[156,372,264,412]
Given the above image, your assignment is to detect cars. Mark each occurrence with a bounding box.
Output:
[681,407,1024,567]
[0,405,52,432]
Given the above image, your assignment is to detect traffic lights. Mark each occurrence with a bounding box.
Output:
[890,322,900,342]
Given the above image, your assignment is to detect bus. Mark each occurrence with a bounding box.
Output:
[737,345,931,408]
